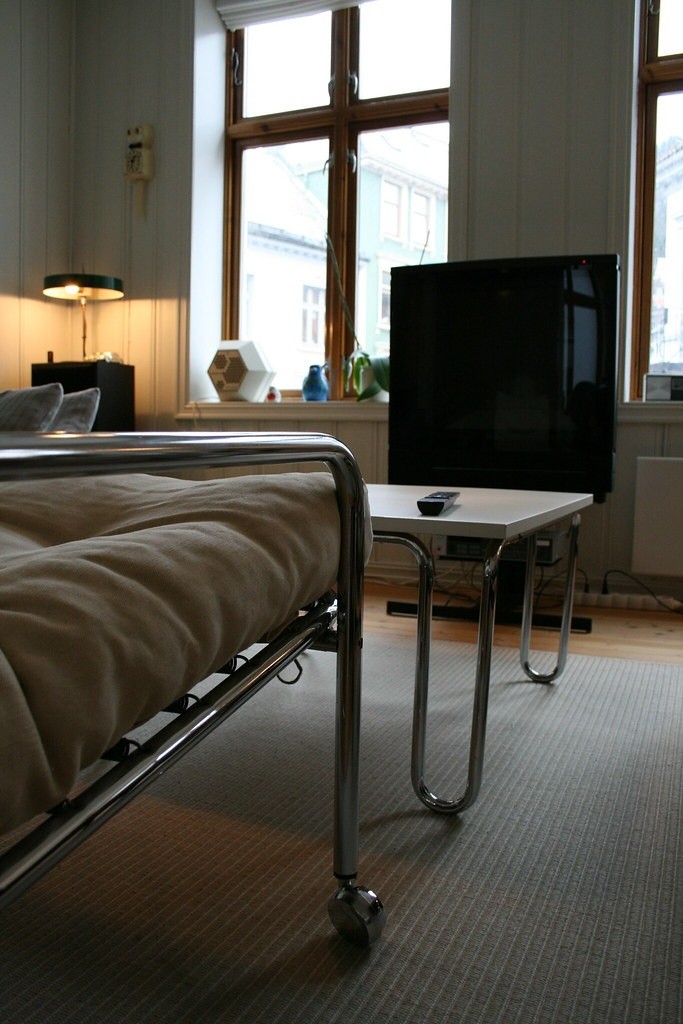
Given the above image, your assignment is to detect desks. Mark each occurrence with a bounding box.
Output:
[366,483,597,814]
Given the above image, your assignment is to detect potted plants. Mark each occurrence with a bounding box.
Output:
[324,232,389,404]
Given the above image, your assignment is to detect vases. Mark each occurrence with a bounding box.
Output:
[301,365,330,403]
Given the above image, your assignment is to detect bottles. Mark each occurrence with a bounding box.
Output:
[301,364,330,401]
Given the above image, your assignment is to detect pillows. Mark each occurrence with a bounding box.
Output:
[47,388,101,433]
[1,383,64,433]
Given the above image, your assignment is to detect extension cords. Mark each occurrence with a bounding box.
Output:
[572,591,683,613]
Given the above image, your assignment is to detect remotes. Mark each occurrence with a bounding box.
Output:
[416,492,459,517]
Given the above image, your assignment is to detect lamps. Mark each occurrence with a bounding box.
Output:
[31,273,136,432]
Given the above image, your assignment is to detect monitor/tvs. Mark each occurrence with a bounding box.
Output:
[388,252,617,506]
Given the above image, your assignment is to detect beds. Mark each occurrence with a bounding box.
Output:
[1,429,387,951]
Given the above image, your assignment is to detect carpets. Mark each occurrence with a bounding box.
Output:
[1,634,683,1024]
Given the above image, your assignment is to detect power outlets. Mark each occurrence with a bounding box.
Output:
[433,535,447,555]
